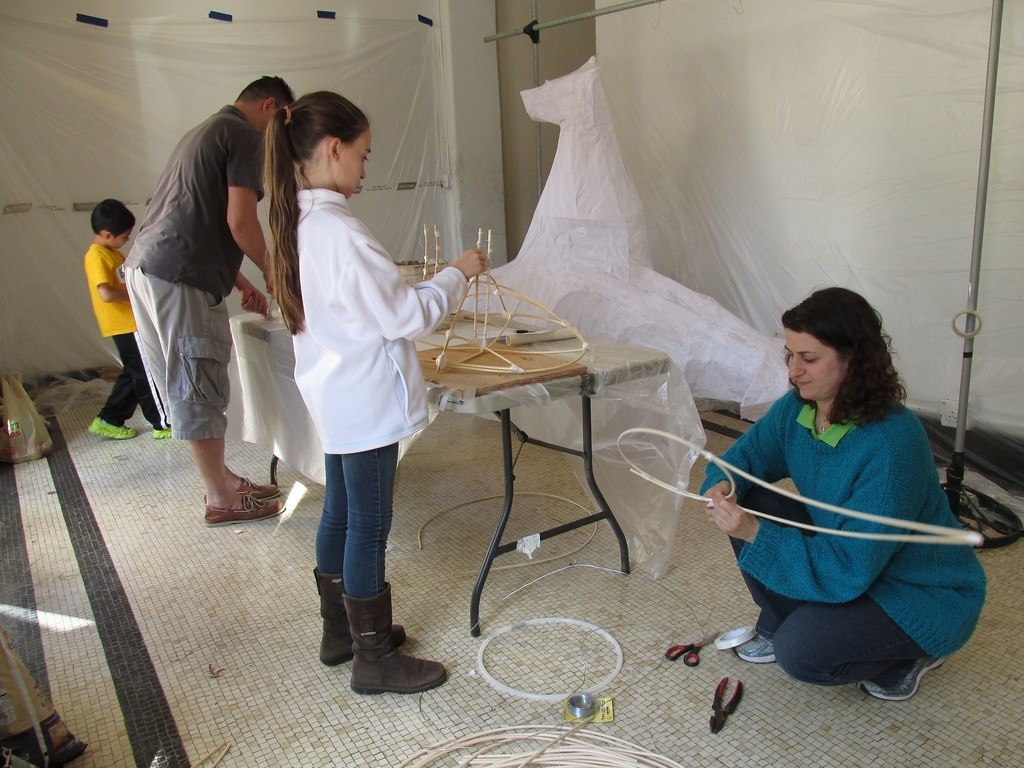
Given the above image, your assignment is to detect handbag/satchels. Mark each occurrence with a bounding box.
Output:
[0,628,88,768]
[0,369,53,464]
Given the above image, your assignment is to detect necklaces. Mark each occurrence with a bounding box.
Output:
[812,409,835,435]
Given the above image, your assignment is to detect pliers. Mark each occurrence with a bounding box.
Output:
[709,676,743,735]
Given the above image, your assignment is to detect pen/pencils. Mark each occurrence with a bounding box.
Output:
[502,329,535,334]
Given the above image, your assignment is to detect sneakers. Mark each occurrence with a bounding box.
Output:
[856,654,947,702]
[153,425,172,439]
[205,493,286,527]
[732,633,776,663]
[203,476,282,505]
[88,416,137,440]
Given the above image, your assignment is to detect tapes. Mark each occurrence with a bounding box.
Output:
[714,625,757,651]
[950,309,983,339]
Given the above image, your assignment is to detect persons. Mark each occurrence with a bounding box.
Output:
[116,77,305,526]
[699,283,987,699]
[267,88,490,697]
[85,199,181,441]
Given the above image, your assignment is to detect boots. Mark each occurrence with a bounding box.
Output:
[313,566,407,666]
[342,580,448,695]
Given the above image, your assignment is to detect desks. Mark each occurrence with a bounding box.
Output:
[229,311,670,637]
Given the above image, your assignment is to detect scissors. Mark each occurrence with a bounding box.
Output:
[665,631,719,667]
